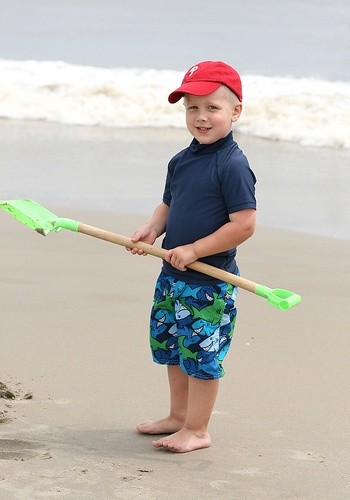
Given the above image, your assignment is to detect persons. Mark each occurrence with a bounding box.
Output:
[126,62,257,453]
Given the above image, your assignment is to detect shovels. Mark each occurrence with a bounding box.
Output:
[0,198,302,311]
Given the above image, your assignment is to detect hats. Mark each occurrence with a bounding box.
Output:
[168,61,242,104]
